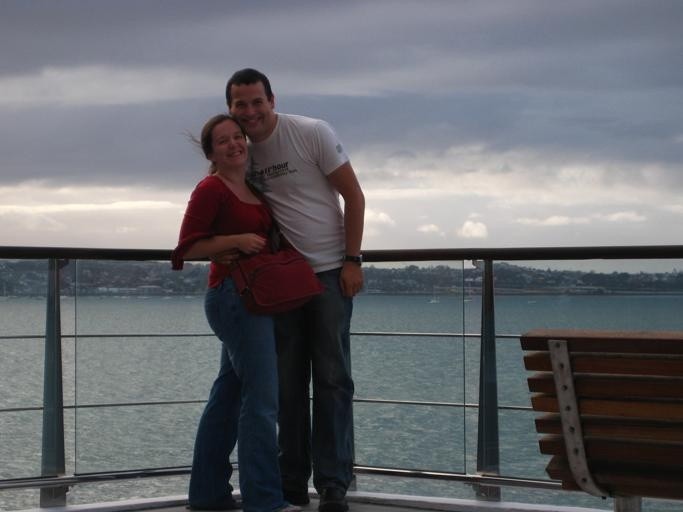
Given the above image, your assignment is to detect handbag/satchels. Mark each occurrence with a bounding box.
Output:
[230,243,324,315]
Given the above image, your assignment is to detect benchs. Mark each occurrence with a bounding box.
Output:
[520,331,682,512]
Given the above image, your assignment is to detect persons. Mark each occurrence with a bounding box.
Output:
[208,66,365,511]
[171,111,302,511]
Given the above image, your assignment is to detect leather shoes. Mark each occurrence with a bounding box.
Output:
[282,490,310,511]
[317,487,349,512]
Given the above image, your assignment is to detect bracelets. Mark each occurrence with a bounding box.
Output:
[341,253,363,266]
